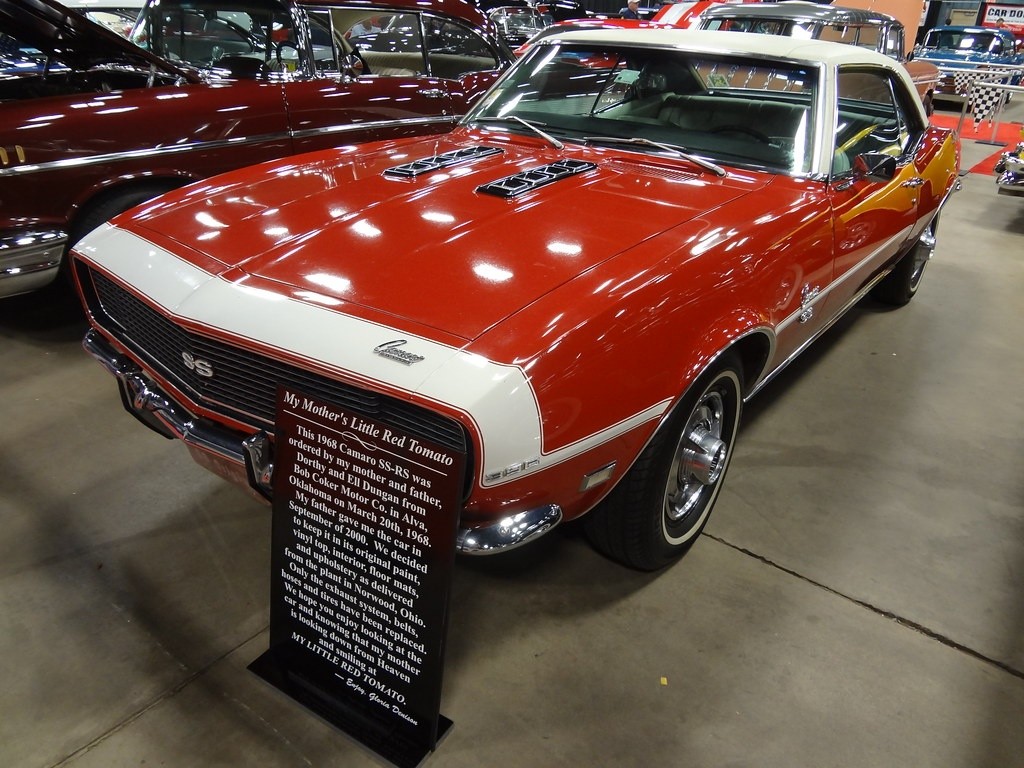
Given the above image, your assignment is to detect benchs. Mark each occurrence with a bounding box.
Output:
[659,93,899,176]
[359,51,494,81]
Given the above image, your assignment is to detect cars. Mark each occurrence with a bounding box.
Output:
[69,0,666,92]
[679,1,942,128]
[64,20,961,575]
[910,26,1024,108]
[1,0,518,328]
